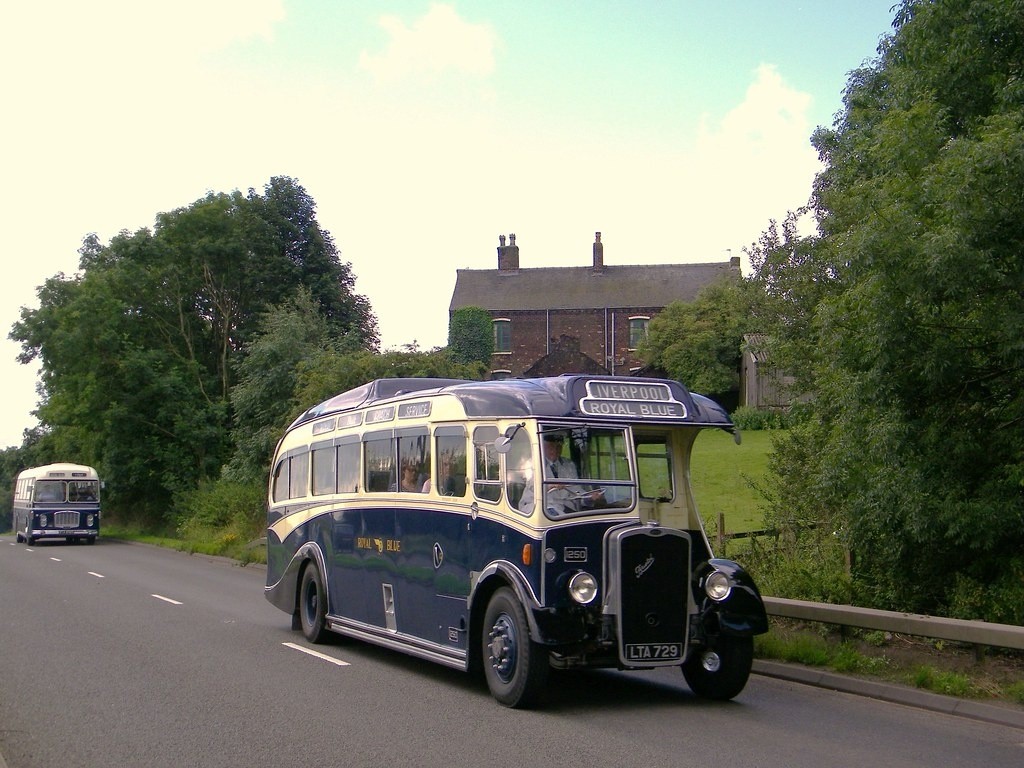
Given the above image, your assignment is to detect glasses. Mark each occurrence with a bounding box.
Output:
[406,467,419,473]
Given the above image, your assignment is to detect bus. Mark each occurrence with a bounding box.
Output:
[12,462,106,546]
[263,372,771,709]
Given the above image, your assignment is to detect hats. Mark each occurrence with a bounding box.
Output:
[542,430,567,443]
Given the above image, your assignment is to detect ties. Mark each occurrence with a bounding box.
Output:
[550,465,558,478]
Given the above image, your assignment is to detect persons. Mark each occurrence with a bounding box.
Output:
[385,453,458,495]
[37,485,54,502]
[518,432,606,516]
[82,486,97,500]
